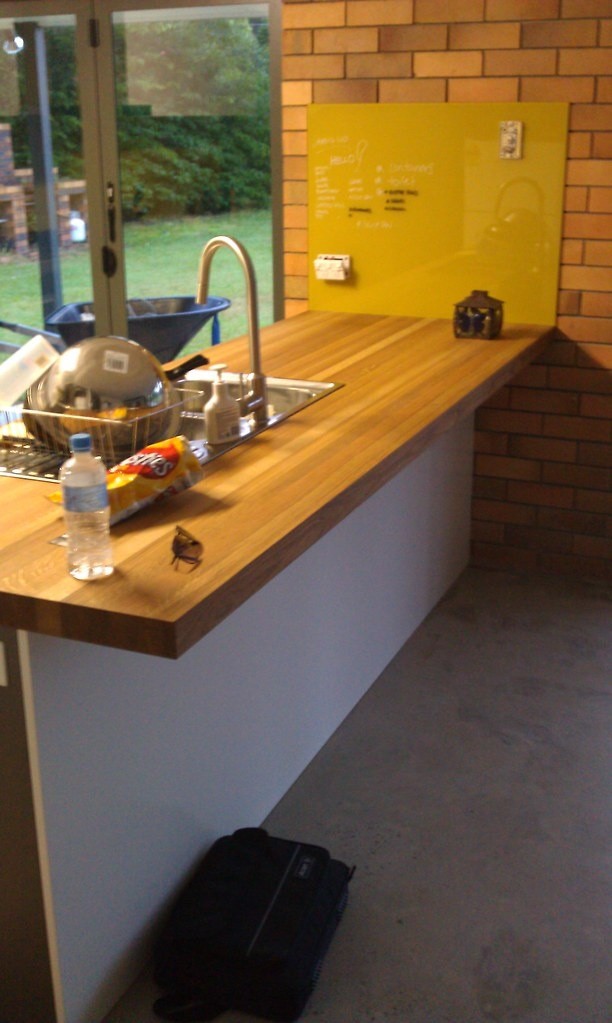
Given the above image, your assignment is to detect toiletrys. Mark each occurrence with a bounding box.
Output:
[203,364,241,444]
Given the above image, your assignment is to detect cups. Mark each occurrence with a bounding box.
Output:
[0,335,60,409]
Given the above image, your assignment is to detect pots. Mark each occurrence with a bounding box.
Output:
[22,336,209,457]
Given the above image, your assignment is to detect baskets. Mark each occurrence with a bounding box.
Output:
[0,386,205,485]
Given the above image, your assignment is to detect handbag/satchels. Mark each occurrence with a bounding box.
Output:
[152,827,356,1023]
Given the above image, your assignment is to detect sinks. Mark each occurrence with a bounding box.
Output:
[174,368,346,423]
[172,413,251,464]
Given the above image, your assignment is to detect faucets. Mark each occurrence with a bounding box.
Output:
[194,235,269,426]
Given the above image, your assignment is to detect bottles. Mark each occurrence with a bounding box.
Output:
[58,433,115,583]
